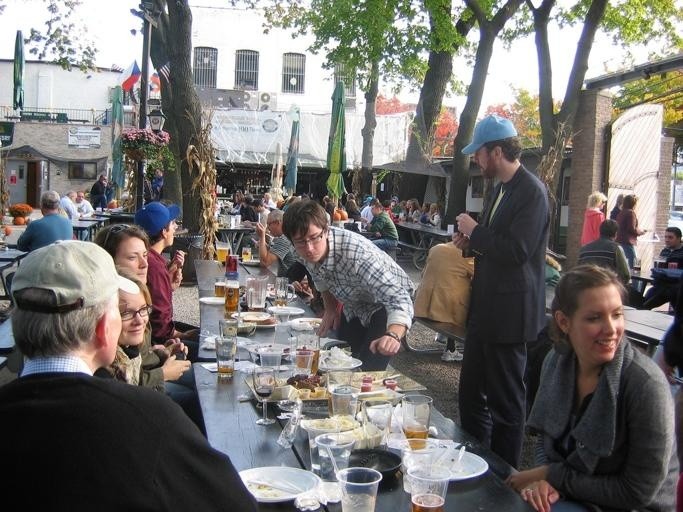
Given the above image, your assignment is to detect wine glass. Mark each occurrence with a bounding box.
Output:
[250,364,277,425]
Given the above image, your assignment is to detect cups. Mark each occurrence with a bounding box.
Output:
[394,210,456,236]
[212,243,321,379]
[668,262,677,272]
[402,440,435,495]
[306,417,354,503]
[402,395,432,448]
[298,374,397,399]
[406,465,451,511]
[217,186,227,194]
[632,257,641,272]
[335,466,382,512]
[96,207,109,214]
[361,399,392,446]
[326,369,361,420]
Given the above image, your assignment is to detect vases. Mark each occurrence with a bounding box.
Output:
[15,216,25,225]
[124,147,160,162]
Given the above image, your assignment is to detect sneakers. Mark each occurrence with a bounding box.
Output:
[435,332,447,345]
[440,349,463,361]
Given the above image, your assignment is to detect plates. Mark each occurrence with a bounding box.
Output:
[294,317,325,327]
[197,296,225,305]
[270,292,297,299]
[299,412,384,450]
[405,448,488,481]
[347,447,400,474]
[315,353,363,373]
[267,305,303,316]
[237,466,324,502]
[241,369,428,402]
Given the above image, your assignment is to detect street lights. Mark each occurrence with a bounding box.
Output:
[130,0,170,215]
[146,107,167,202]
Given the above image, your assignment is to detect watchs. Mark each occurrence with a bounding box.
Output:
[385,331,400,343]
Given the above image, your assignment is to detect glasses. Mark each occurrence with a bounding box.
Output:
[293,228,323,248]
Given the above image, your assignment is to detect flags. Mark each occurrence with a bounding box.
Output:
[118,59,141,92]
[149,73,160,93]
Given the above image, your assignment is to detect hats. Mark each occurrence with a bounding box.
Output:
[461,113,518,155]
[10,190,180,313]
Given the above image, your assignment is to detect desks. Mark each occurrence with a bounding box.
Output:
[194,259,294,292]
[192,362,577,512]
[197,290,344,360]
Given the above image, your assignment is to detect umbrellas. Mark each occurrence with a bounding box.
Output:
[326,80,348,207]
[111,87,125,200]
[12,30,25,123]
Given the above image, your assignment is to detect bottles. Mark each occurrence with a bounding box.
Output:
[218,216,222,226]
[235,214,240,224]
[71,208,78,225]
[240,170,270,187]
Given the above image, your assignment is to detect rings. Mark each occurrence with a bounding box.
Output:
[527,488,532,492]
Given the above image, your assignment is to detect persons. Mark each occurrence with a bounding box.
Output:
[5,190,73,312]
[452,114,552,469]
[576,190,648,285]
[545,253,562,287]
[92,202,206,431]
[60,192,81,220]
[322,190,443,252]
[0,238,260,512]
[233,190,326,316]
[282,200,417,372]
[638,226,682,312]
[90,175,118,210]
[75,191,95,241]
[503,265,680,511]
[412,231,474,361]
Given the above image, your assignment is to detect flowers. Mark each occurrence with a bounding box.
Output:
[122,129,175,178]
[9,204,33,217]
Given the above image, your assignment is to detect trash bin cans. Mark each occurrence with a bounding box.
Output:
[170,233,205,287]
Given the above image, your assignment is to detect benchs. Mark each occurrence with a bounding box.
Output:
[398,241,427,270]
[0,315,17,370]
[401,313,466,355]
[377,363,427,392]
[383,247,401,263]
[399,240,427,251]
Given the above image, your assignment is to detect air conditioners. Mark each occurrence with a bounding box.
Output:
[259,92,276,111]
[244,92,259,111]
